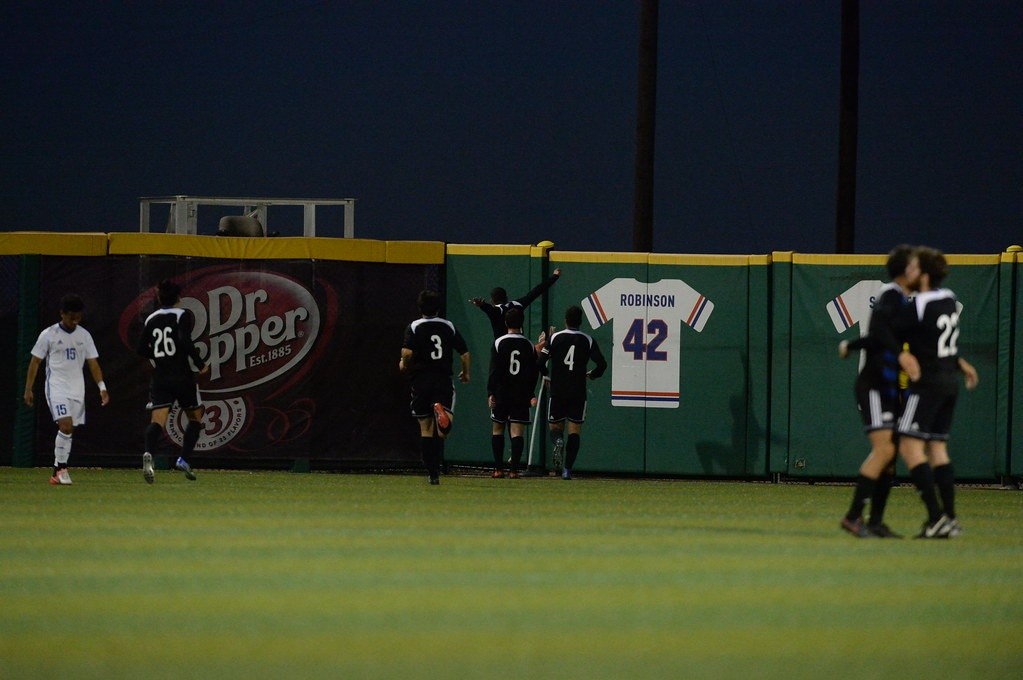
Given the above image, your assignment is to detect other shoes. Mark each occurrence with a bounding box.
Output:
[428,476,439,485]
[553,438,564,469]
[492,471,505,478]
[434,402,449,428]
[562,466,572,480]
[509,471,519,479]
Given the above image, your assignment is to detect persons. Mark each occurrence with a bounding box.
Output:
[469,267,561,374]
[839,244,978,538]
[539,307,606,479]
[142,281,208,483]
[488,308,538,478]
[400,291,470,485]
[24,293,109,485]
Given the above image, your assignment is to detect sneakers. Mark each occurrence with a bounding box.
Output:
[842,515,873,537]
[176,457,196,480]
[56,465,73,484]
[867,518,896,537]
[916,513,963,539]
[50,475,60,484]
[143,452,154,484]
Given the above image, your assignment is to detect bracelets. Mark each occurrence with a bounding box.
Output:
[98,381,106,391]
